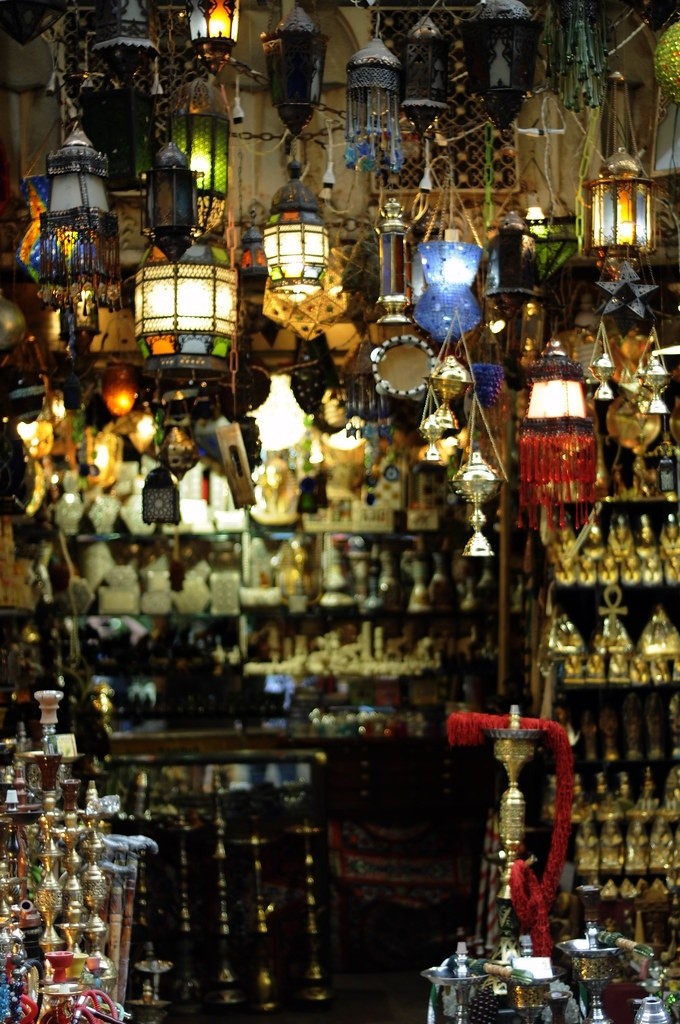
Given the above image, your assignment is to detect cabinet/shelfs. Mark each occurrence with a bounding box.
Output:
[0,495,510,725]
[523,493,680,914]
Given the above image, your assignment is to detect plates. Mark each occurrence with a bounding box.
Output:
[16,751,84,763]
[4,811,43,825]
[40,983,82,996]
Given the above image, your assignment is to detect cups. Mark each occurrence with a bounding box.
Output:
[421,966,502,1024]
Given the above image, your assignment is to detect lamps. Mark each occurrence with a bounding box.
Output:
[0,0,680,532]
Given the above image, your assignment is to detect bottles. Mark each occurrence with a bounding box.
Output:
[544,991,573,1024]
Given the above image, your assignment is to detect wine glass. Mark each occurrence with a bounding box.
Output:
[556,939,620,1024]
[510,965,566,1024]
